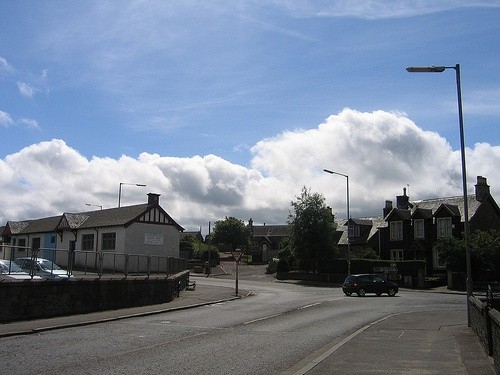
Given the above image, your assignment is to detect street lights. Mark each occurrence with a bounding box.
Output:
[86,203,102,210]
[323,169,350,275]
[406,64,474,297]
[118,182,146,209]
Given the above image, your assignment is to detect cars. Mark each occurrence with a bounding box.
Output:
[15,258,75,279]
[1,260,43,281]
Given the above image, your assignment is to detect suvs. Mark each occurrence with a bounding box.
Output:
[343,273,399,297]
